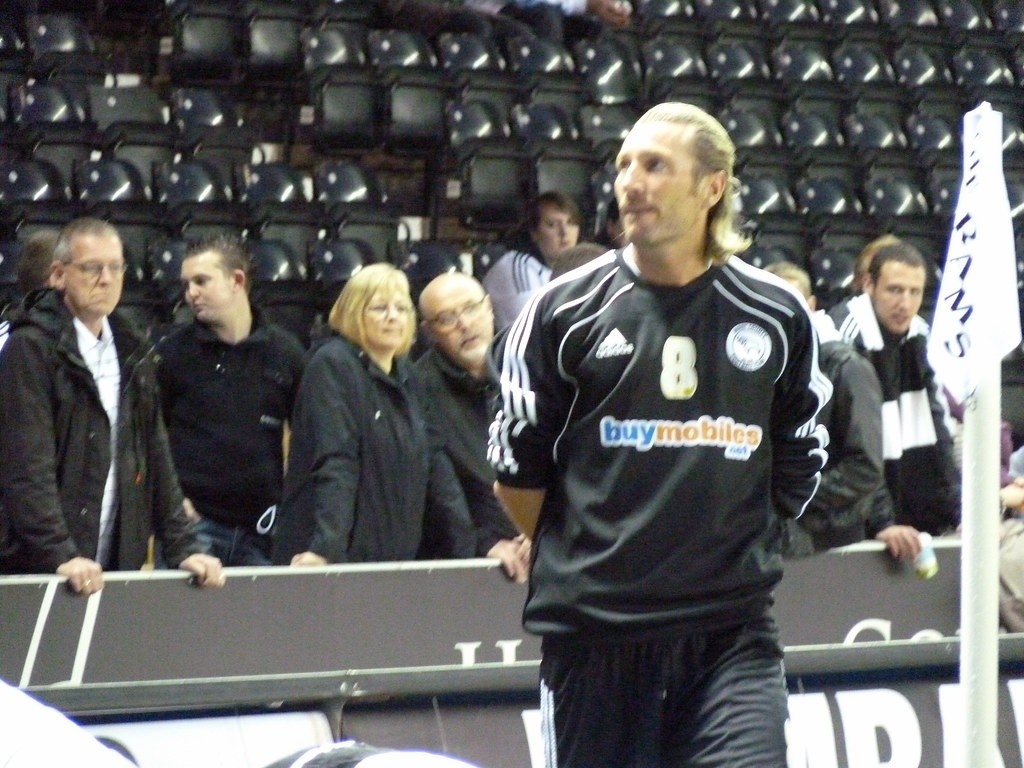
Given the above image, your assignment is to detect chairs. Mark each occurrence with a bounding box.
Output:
[0,0,1024,454]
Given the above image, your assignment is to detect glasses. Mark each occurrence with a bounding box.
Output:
[428,295,486,333]
[68,261,128,277]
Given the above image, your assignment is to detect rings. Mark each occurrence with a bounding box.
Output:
[82,579,91,586]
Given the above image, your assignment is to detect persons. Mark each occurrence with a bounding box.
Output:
[515,0,628,37]
[483,189,581,333]
[492,243,610,412]
[0,218,226,595]
[760,262,882,559]
[829,236,963,540]
[149,232,303,570]
[411,270,532,584]
[267,261,429,565]
[594,198,629,249]
[486,101,834,768]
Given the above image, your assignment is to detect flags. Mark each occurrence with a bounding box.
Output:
[925,110,1022,406]
